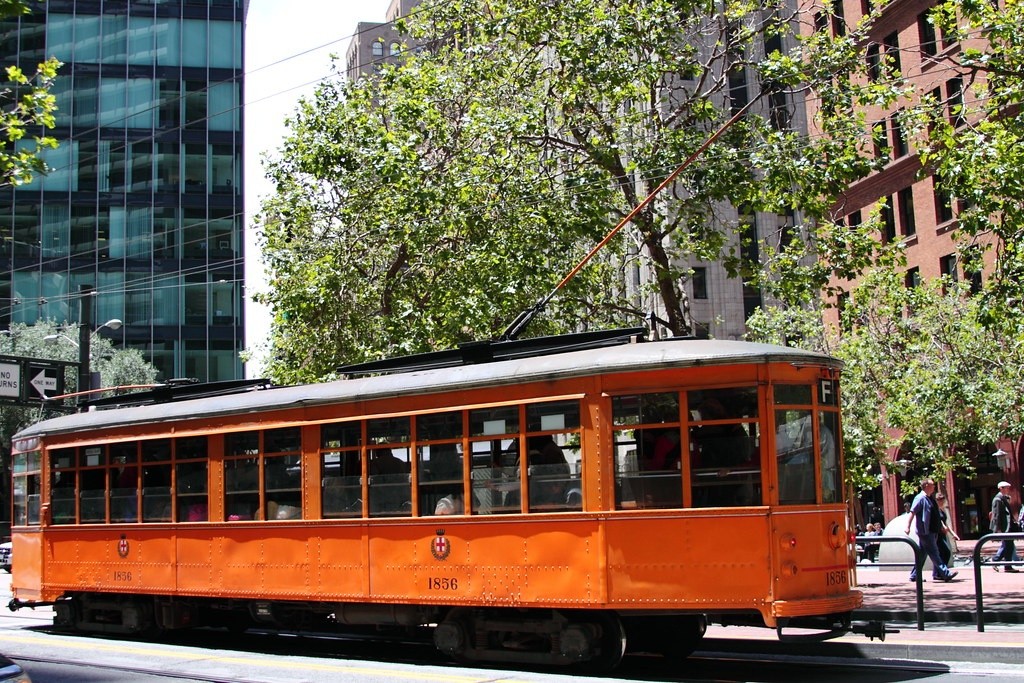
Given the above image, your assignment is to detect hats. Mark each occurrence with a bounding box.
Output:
[998,482,1012,489]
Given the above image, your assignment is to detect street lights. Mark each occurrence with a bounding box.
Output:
[897,458,912,502]
[993,449,1008,482]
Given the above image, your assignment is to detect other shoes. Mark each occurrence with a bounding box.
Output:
[933,577,946,582]
[988,558,999,572]
[911,578,926,582]
[1004,568,1020,572]
[944,571,958,582]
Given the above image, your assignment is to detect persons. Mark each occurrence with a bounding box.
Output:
[855,523,883,563]
[989,482,1024,572]
[52,433,362,522]
[492,427,570,506]
[634,399,835,507]
[905,478,960,582]
[370,436,464,515]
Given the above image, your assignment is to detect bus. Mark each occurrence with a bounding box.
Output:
[9,327,901,678]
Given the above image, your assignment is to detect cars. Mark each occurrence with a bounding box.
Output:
[0,542,13,574]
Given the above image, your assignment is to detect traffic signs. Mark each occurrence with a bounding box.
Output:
[29,367,58,399]
